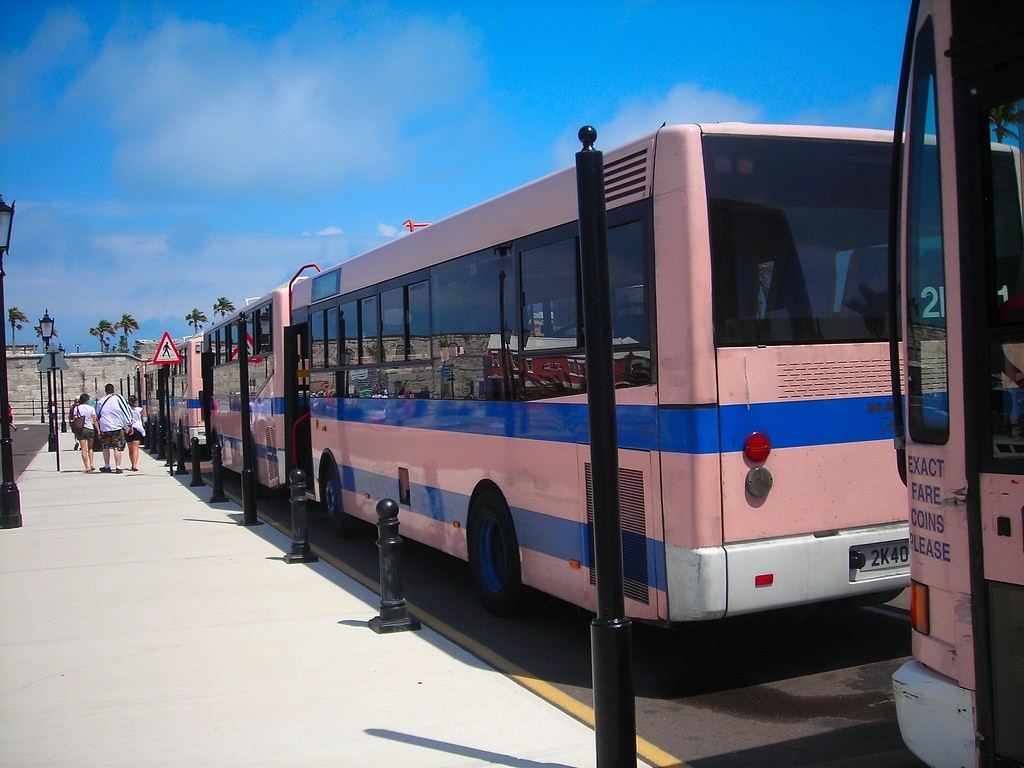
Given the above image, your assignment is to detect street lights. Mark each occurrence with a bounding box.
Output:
[0,194,22,531]
[38,308,59,452]
[58,342,67,432]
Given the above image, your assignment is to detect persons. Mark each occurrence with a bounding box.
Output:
[96,384,134,474]
[309,381,431,422]
[70,393,101,472]
[8,403,17,431]
[122,395,146,471]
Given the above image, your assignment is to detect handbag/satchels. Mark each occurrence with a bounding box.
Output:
[70,404,85,432]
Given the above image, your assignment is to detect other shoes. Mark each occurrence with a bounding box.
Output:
[131,468,139,472]
[90,466,96,471]
[99,467,111,473]
[86,470,91,473]
[14,427,18,431]
[115,468,125,474]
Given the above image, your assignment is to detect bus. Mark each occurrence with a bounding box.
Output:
[133,328,207,455]
[203,121,1020,638]
[882,0,1024,768]
